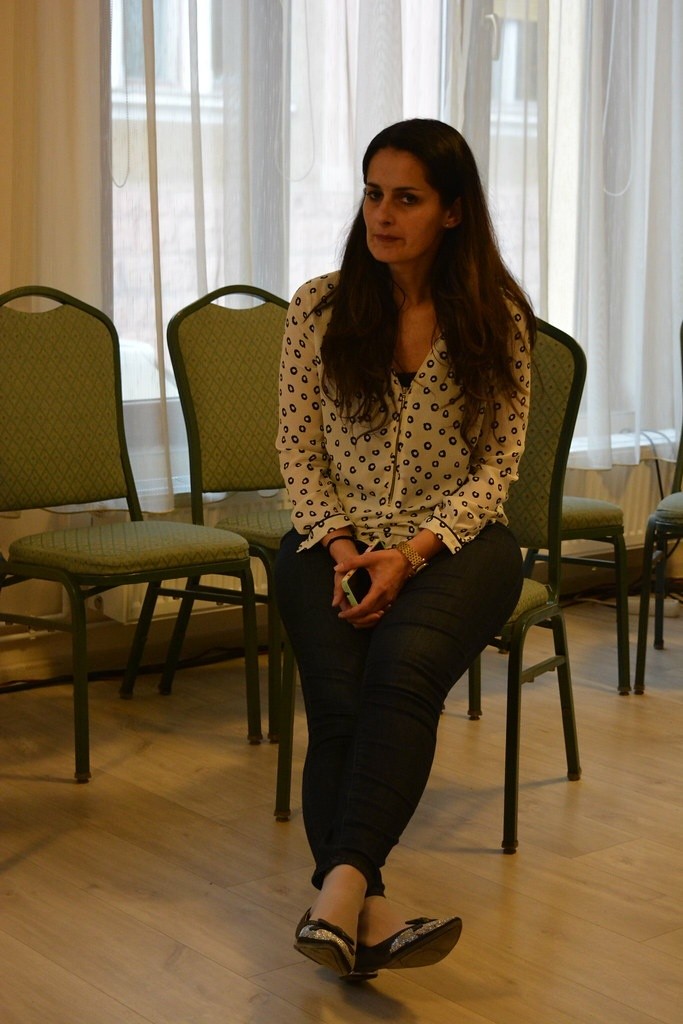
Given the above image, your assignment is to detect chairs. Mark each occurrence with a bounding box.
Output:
[156,285,291,744]
[467,495,632,720]
[634,323,683,695]
[274,315,587,855]
[0,285,264,783]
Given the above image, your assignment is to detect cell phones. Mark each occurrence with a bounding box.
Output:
[341,539,385,608]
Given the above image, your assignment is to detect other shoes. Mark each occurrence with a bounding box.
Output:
[340,915,462,980]
[293,907,354,976]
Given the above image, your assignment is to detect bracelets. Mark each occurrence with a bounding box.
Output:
[394,541,430,574]
[327,536,358,550]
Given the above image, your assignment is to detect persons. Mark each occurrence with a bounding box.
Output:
[270,118,538,983]
